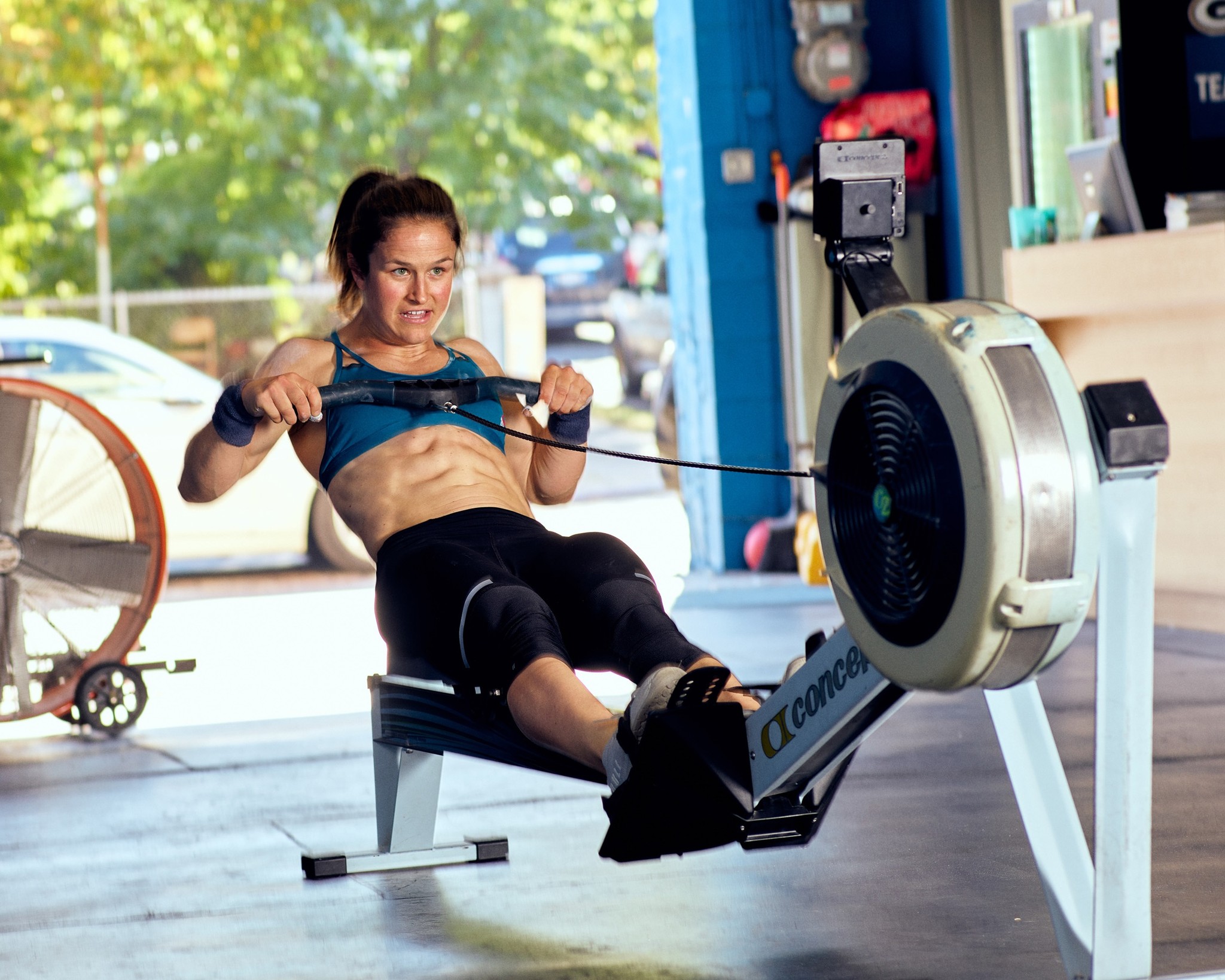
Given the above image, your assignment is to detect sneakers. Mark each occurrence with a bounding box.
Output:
[600,667,694,795]
[782,655,808,685]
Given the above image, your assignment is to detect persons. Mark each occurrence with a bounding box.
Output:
[181,169,810,804]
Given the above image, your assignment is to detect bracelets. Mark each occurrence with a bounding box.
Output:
[212,378,264,448]
[548,401,592,446]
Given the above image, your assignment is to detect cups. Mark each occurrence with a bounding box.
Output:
[1007,206,1057,248]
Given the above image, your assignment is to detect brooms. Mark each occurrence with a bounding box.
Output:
[757,151,805,573]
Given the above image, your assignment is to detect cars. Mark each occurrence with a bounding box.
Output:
[0,318,377,577]
[491,208,679,500]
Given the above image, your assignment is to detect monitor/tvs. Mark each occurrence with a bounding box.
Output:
[1066,136,1145,241]
